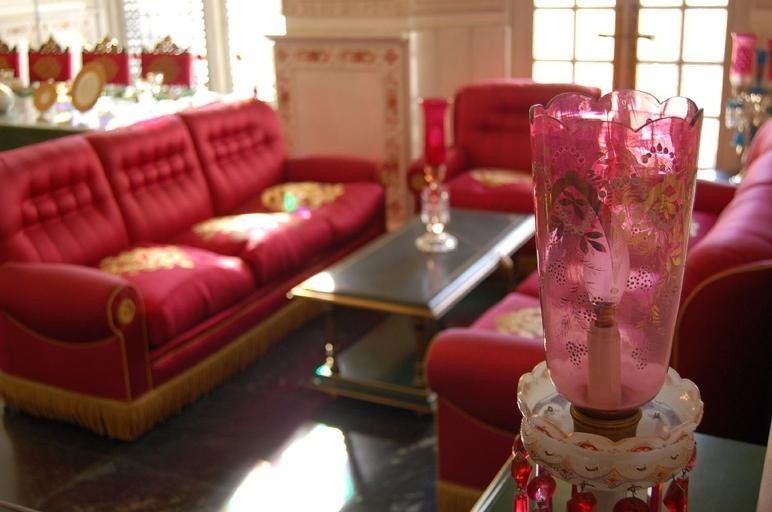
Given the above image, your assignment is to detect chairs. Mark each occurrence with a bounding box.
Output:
[0,34,196,91]
